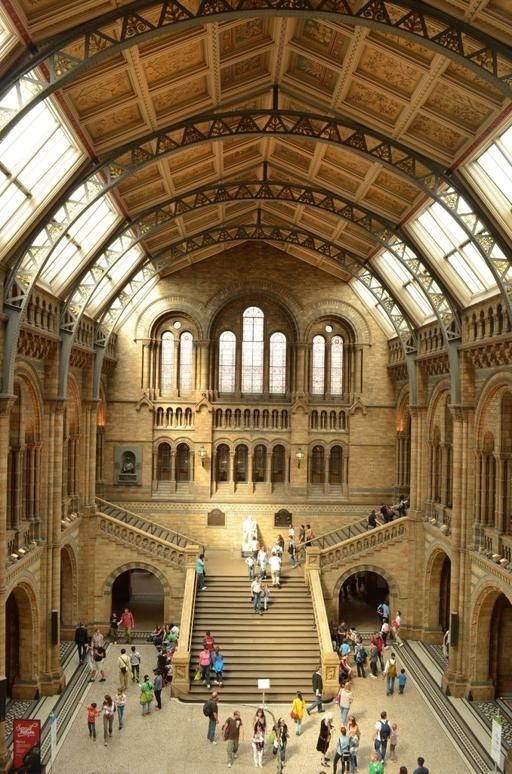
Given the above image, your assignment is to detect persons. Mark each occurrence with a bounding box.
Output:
[245,525,312,614]
[110,608,134,645]
[197,554,207,590]
[442,628,449,665]
[207,692,288,769]
[292,665,429,774]
[118,624,179,716]
[76,623,106,682]
[332,601,406,696]
[369,495,408,528]
[199,632,223,688]
[88,688,125,746]
[342,570,366,595]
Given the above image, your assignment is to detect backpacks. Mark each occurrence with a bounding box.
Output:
[388,660,397,678]
[379,720,390,741]
[202,701,218,717]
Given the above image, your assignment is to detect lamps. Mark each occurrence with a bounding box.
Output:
[295,446,305,470]
[196,444,208,467]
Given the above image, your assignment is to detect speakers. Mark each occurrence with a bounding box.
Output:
[451,612,458,647]
[51,610,58,644]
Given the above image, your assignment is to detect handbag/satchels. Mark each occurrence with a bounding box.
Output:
[213,659,225,674]
[121,667,127,673]
[343,751,351,762]
[290,710,299,720]
[335,688,342,704]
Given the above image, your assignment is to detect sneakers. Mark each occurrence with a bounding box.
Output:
[386,690,394,696]
[305,709,311,716]
[87,678,95,683]
[109,732,114,738]
[99,678,106,682]
[320,762,331,768]
[208,738,218,745]
[317,708,326,713]
[369,672,378,678]
[397,643,405,648]
[233,753,238,759]
[321,757,331,762]
[227,762,232,768]
[254,762,262,769]
[104,740,109,746]
[198,586,207,590]
